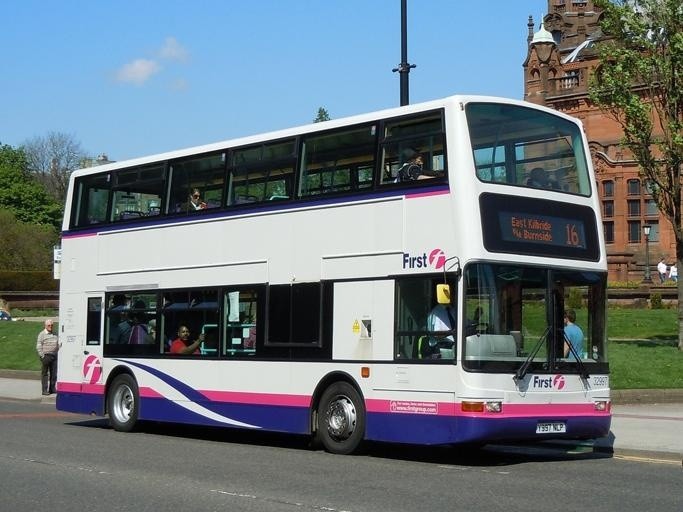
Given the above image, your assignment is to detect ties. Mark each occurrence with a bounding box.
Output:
[446,307,455,330]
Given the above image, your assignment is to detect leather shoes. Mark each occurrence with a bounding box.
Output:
[42,391,57,395]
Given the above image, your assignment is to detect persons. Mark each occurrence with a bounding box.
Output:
[396,153,437,183]
[36,319,58,395]
[179,188,207,212]
[238,328,257,349]
[657,258,668,285]
[427,302,483,354]
[563,309,584,359]
[668,262,678,282]
[112,300,148,344]
[526,168,561,189]
[169,324,207,354]
[107,294,131,340]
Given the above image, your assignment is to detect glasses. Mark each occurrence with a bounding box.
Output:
[191,193,201,199]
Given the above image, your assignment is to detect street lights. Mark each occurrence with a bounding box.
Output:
[641,224,652,284]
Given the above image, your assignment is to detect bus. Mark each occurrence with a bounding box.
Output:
[55,93,612,453]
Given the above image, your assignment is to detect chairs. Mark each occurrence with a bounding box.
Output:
[82,194,258,223]
